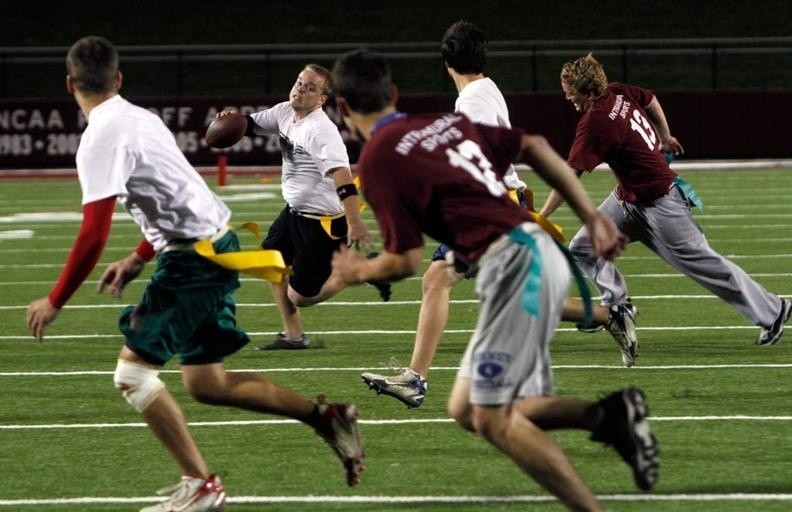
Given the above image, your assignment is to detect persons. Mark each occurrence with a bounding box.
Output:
[26,36,366,512]
[537,52,791,367]
[205,63,393,352]
[331,46,662,512]
[358,18,640,411]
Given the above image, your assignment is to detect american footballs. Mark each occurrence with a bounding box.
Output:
[206,114,246,149]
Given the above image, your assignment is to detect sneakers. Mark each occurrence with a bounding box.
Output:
[313,394,365,487]
[583,385,661,491]
[361,366,429,408]
[136,472,226,512]
[574,298,638,332]
[253,331,310,352]
[363,251,392,302]
[604,300,640,369]
[756,297,792,347]
